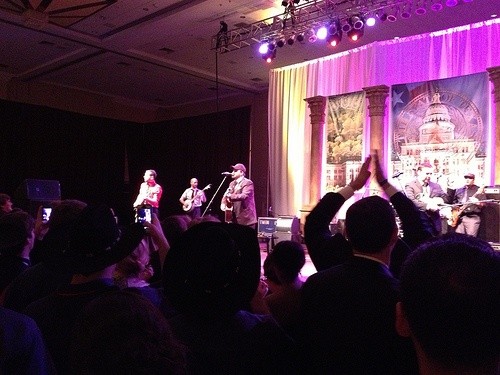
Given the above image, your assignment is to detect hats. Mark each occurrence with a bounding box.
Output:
[162,222,260,313]
[72,205,144,272]
[464,174,474,178]
[232,163,246,172]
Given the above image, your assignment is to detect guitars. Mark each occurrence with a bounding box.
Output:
[183,184,211,213]
[225,188,233,223]
[446,185,484,227]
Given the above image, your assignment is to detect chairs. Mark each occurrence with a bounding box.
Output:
[254,216,278,255]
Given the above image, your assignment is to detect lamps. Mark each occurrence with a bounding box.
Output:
[258,0,444,64]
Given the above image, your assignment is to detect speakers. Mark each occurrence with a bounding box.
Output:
[477,204,500,243]
[24,179,61,200]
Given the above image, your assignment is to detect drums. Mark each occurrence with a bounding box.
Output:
[272,215,302,246]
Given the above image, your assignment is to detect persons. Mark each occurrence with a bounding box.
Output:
[0,192,188,375]
[392,159,451,194]
[287,195,420,375]
[221,164,258,230]
[395,232,500,375]
[133,170,163,224]
[162,221,296,375]
[179,178,207,220]
[263,241,309,320]
[303,149,435,280]
[447,173,487,237]
[404,166,450,234]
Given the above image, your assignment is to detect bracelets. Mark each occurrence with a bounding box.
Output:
[380,179,388,187]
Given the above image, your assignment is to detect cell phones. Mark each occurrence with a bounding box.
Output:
[41,207,52,223]
[134,206,152,233]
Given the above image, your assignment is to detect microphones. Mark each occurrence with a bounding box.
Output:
[392,172,403,178]
[221,173,232,175]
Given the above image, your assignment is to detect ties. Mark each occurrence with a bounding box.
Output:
[192,191,194,203]
